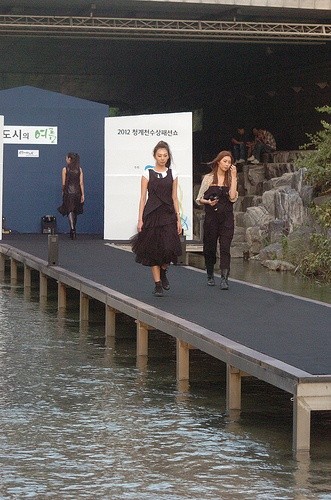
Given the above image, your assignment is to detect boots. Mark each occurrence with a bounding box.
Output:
[206,266,215,286]
[220,268,229,289]
[153,281,163,296]
[160,269,170,290]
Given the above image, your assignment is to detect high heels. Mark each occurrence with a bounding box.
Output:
[71,229,77,239]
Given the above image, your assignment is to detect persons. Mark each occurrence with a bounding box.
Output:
[231,125,248,165]
[196,151,239,290]
[130,141,182,297]
[247,127,276,164]
[57,152,85,239]
